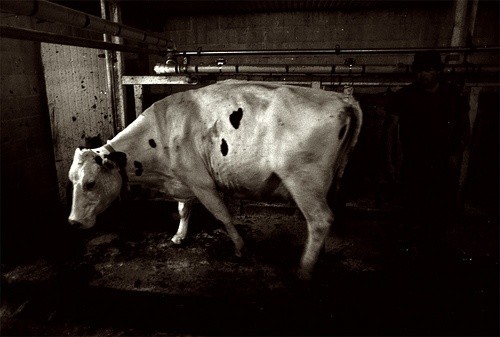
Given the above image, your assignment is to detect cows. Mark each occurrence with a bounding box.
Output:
[67,78,362,280]
